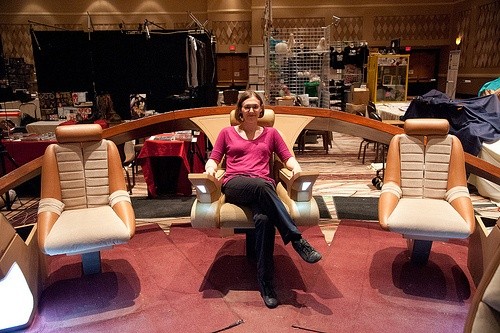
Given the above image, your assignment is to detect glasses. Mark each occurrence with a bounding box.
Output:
[242,105,260,111]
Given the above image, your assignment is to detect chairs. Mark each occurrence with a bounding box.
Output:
[185,107,320,268]
[295,96,332,156]
[377,117,476,267]
[33,123,135,277]
[219,89,239,105]
[358,99,390,165]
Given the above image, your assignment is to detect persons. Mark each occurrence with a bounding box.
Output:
[205,91,321,308]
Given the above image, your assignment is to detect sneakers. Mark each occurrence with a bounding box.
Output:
[258,280,278,306]
[292,238,322,263]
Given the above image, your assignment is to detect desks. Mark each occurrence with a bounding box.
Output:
[138,130,196,198]
[3,133,58,165]
